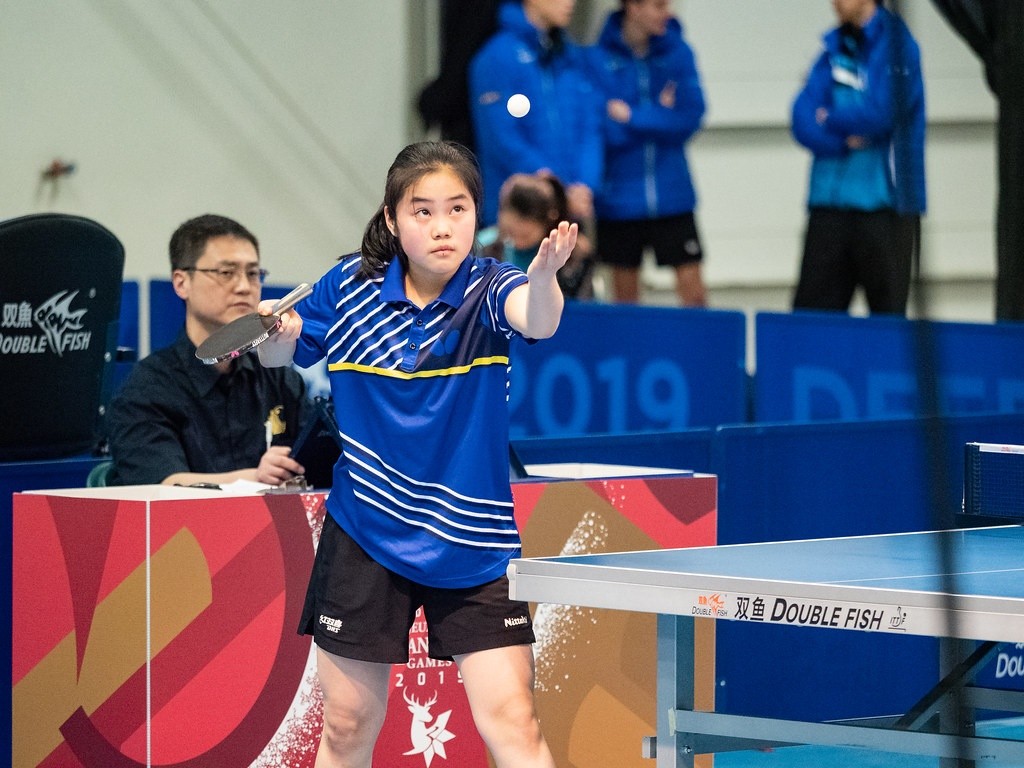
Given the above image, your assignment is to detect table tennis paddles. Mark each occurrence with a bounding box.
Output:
[195,282,313,365]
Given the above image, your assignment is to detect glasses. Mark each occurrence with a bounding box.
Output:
[183,265,267,285]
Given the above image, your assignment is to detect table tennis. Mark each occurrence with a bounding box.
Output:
[506,93,531,119]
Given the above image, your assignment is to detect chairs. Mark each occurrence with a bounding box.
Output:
[0,213,137,464]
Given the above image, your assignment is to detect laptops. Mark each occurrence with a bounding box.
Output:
[282,397,343,488]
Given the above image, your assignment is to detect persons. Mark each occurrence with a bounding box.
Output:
[103,212,340,487]
[467,0,608,304]
[584,0,712,308]
[786,0,928,319]
[253,138,581,768]
[466,167,599,303]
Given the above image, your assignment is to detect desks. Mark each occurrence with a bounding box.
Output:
[14,463,719,768]
[506,442,1023,768]
